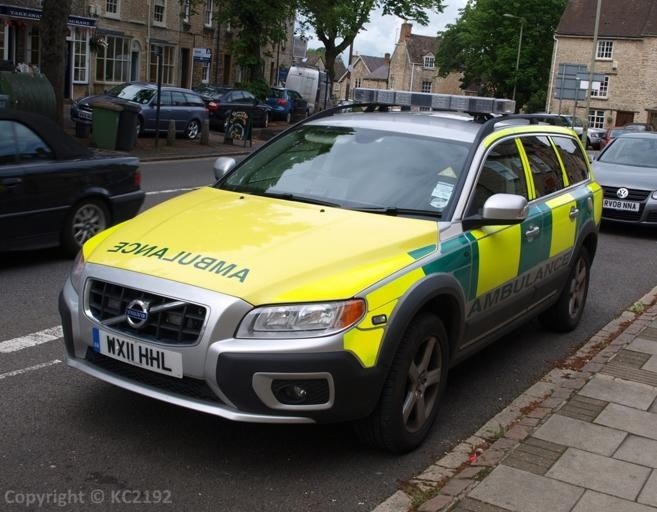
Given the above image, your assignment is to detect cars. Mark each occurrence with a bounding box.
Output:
[0,104,148,261]
[68,79,312,140]
[588,120,657,234]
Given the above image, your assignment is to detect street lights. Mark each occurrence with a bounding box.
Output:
[503,13,525,99]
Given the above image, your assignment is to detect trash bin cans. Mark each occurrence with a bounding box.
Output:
[87,98,142,150]
